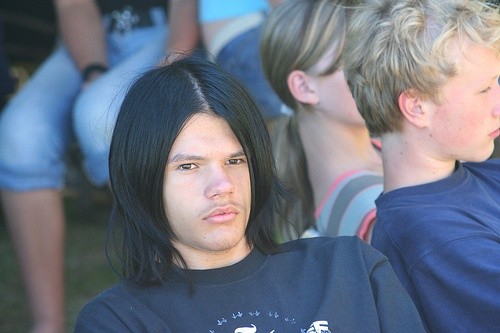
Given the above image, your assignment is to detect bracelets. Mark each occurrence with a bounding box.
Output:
[82,63,109,83]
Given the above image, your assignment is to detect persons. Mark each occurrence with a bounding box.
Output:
[0,0,197,333]
[72,59,427,333]
[199,0,382,246]
[342,0,500,333]
